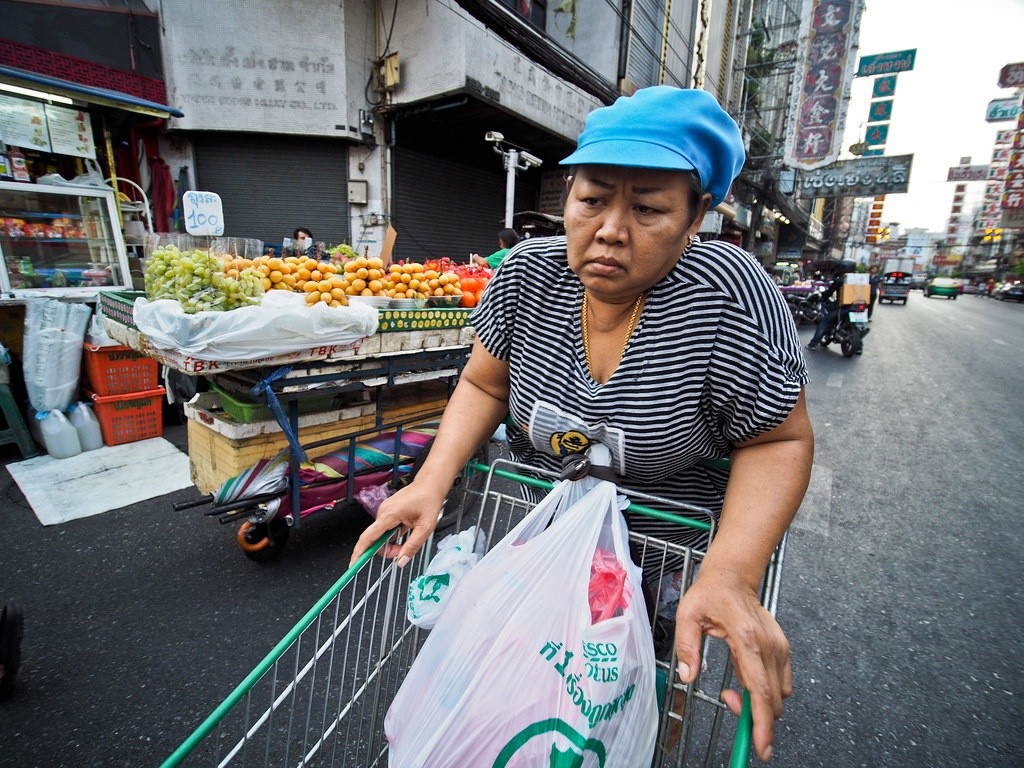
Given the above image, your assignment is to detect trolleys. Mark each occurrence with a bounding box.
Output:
[158,459,788,768]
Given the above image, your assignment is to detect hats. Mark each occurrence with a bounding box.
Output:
[841,261,855,273]
[558,84,746,210]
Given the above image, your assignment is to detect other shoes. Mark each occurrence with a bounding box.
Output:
[856,349,862,354]
[805,342,816,350]
[869,319,872,321]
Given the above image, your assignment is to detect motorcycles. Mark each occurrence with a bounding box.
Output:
[877,256,916,305]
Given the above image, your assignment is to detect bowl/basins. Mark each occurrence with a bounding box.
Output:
[348,296,391,309]
[388,298,428,309]
[427,296,461,308]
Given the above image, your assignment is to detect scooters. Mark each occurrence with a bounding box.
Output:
[776,259,837,330]
[808,286,872,358]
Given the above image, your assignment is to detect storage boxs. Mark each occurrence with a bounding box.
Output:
[842,272,870,285]
[838,285,870,307]
[80,327,475,447]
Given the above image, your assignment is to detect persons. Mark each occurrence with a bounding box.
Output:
[976,279,994,298]
[801,261,856,351]
[349,85,815,768]
[867,265,882,325]
[795,258,816,279]
[294,227,312,243]
[474,228,520,269]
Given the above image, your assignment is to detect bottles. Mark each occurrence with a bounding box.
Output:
[41,408,82,459]
[69,401,104,451]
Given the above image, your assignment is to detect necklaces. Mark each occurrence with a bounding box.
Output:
[575,285,642,373]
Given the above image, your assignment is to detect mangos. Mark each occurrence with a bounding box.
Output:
[218,253,462,309]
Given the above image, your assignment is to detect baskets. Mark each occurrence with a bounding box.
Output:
[213,383,336,423]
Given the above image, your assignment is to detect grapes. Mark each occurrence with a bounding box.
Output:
[327,243,352,257]
[145,243,266,314]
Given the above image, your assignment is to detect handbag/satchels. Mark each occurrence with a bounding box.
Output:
[382,444,660,768]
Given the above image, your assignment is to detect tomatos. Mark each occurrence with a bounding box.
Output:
[460,277,488,308]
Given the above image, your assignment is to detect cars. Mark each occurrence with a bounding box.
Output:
[910,271,1024,303]
